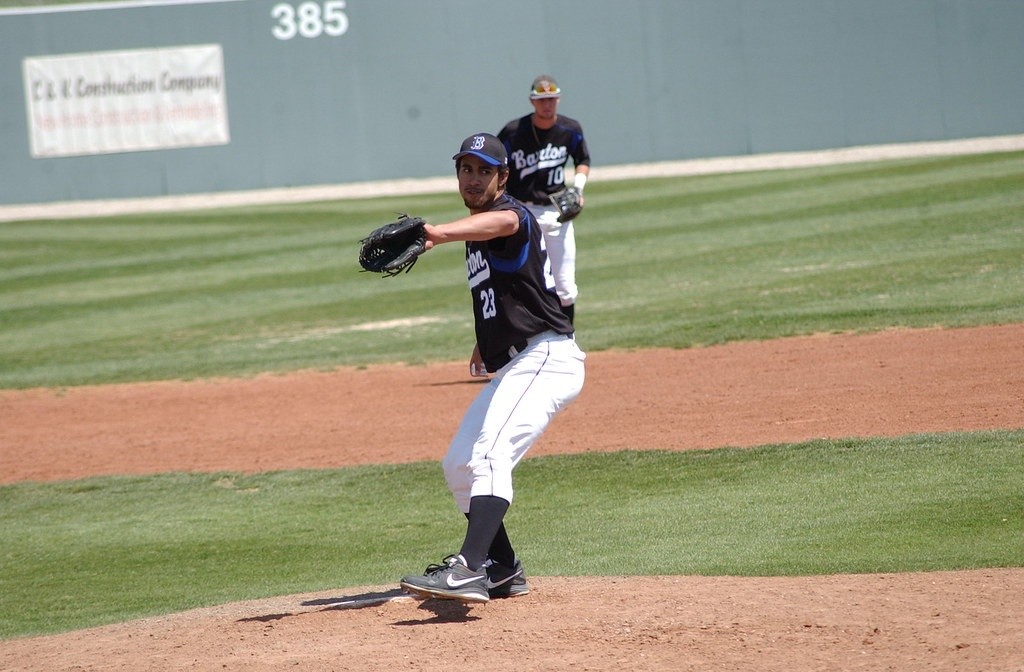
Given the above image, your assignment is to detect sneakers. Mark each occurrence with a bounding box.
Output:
[426,559,529,597]
[399,556,489,608]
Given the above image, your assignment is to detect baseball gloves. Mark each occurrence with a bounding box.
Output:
[555,187,583,222]
[357,212,426,278]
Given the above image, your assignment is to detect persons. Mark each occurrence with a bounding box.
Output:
[394,133,587,605]
[495,73,592,348]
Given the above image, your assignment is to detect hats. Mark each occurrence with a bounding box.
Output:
[529,75,562,101]
[451,133,508,166]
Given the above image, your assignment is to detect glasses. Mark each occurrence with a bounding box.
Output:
[529,86,562,94]
